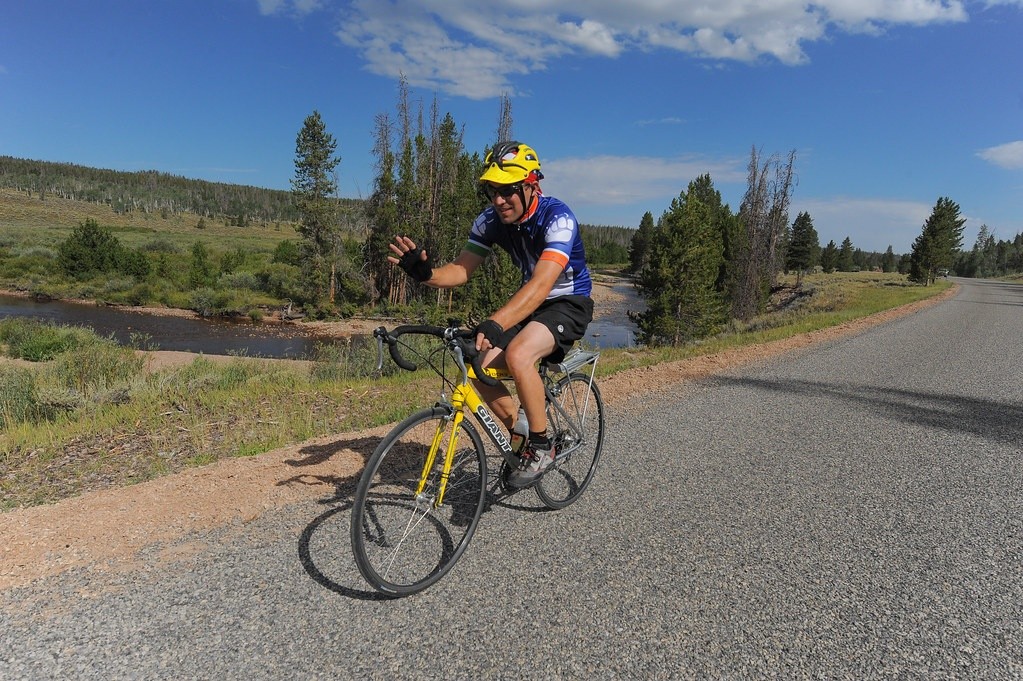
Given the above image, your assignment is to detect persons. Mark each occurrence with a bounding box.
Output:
[384,139,597,491]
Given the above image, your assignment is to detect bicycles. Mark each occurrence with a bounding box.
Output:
[350,315,606,598]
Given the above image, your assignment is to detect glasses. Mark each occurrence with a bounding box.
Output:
[485,184,524,196]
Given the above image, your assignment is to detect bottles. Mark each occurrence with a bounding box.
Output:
[509,406,530,458]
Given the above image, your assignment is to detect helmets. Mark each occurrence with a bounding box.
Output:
[479,141,540,185]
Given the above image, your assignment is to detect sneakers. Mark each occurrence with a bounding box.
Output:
[507,441,556,487]
